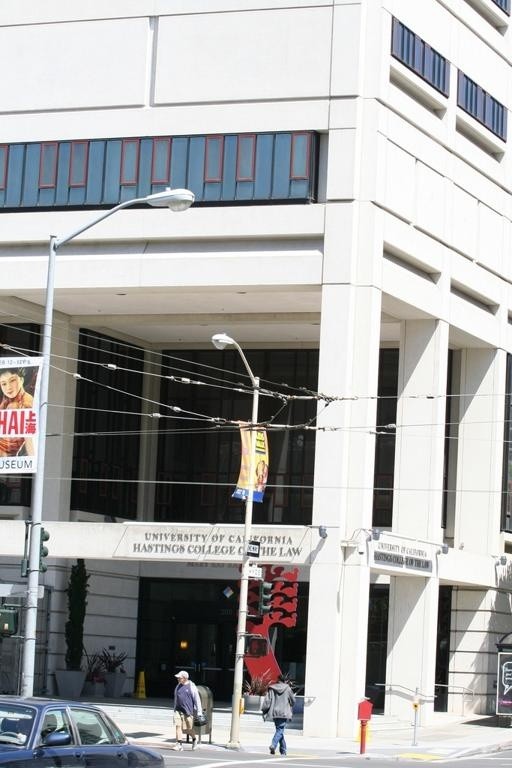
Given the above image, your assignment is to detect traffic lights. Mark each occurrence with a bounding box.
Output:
[0,609,18,635]
[249,636,268,657]
[258,580,274,616]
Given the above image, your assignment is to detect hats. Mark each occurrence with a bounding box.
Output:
[174,670,189,679]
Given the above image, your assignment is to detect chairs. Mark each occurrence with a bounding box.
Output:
[2,715,102,744]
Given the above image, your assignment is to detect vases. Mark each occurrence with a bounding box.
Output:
[292,696,304,714]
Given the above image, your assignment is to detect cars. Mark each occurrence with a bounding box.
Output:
[0,695,165,768]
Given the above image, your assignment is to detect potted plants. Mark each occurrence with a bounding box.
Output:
[55,558,91,697]
[98,648,128,698]
[243,667,271,711]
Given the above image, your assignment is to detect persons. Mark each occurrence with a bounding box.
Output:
[1,367,34,456]
[261,674,296,756]
[171,671,203,750]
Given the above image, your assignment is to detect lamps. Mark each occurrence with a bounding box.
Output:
[350,529,379,540]
[319,526,327,539]
[436,545,448,555]
[495,556,506,566]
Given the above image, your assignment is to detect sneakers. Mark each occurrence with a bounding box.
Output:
[269,745,287,756]
[172,738,198,752]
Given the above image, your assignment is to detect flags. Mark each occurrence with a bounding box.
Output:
[0,356,45,475]
[230,422,270,503]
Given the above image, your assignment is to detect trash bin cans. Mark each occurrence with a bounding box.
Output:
[187,685,214,744]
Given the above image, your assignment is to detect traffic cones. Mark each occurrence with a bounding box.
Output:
[133,671,146,698]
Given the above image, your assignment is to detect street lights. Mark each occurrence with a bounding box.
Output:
[19,189,195,698]
[212,334,261,742]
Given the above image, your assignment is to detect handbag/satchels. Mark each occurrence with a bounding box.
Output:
[194,715,208,727]
